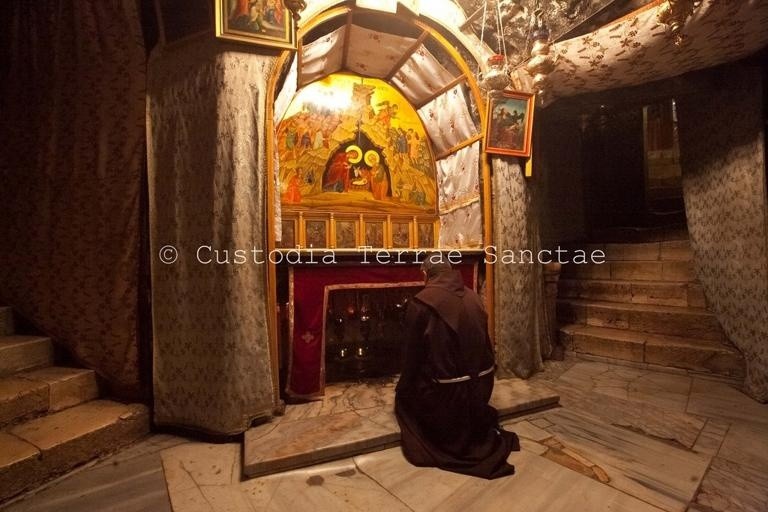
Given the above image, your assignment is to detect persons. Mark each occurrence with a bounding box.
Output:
[495,107,520,149]
[227,0,284,34]
[393,252,521,479]
[277,100,432,207]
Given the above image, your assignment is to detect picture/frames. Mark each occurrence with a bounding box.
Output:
[215,0,299,50]
[155,0,214,49]
[482,90,536,157]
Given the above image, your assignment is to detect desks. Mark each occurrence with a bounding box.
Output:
[275,247,485,400]
